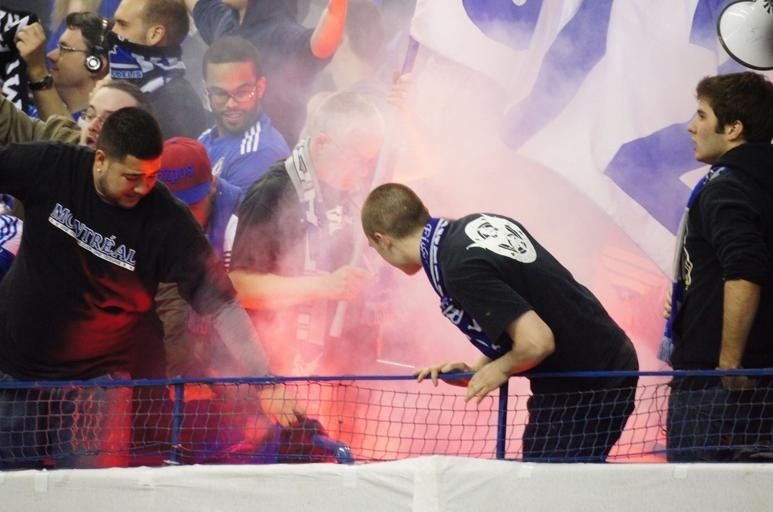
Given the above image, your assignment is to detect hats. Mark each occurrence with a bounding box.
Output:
[158,137,212,207]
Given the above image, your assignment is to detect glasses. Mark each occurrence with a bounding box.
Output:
[81,111,108,127]
[202,84,258,104]
[56,44,93,56]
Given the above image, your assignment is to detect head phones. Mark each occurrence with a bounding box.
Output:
[84,18,108,72]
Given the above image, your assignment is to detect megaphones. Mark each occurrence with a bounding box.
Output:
[714,0,772,71]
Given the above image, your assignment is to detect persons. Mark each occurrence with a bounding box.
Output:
[1,0,414,468]
[656,72,773,463]
[362,183,639,462]
